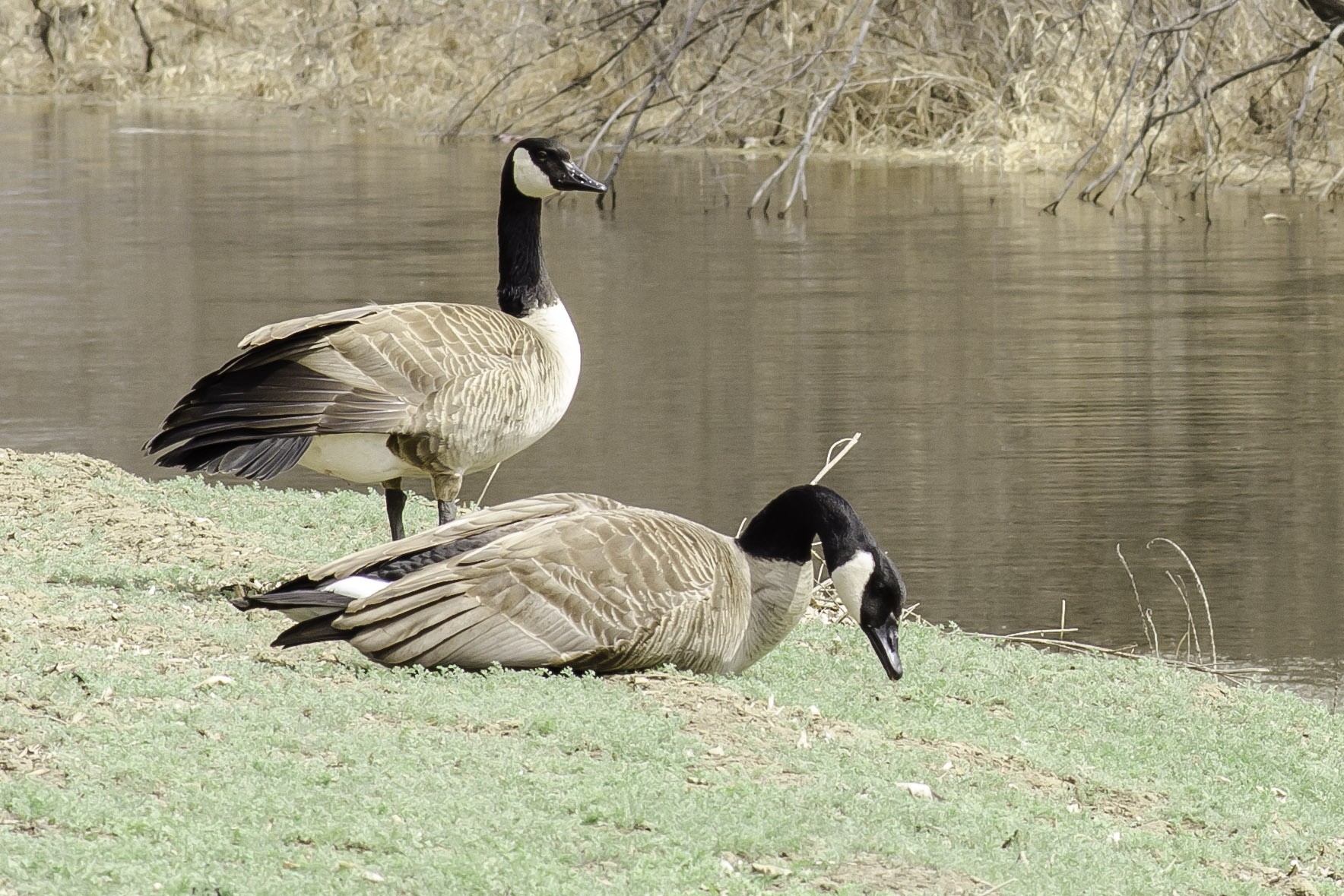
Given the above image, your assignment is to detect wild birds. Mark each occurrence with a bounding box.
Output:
[228,485,906,681]
[143,138,607,538]
